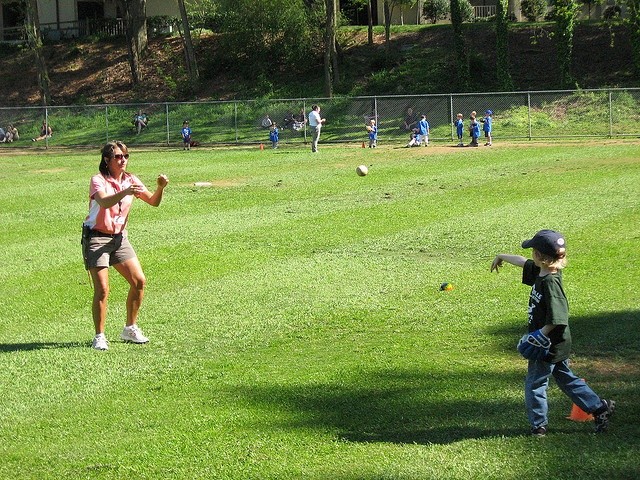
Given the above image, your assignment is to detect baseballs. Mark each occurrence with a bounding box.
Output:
[356,165,369,176]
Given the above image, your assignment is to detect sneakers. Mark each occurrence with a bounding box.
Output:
[527,427,547,438]
[594,399,616,433]
[120,325,149,343]
[93,334,109,350]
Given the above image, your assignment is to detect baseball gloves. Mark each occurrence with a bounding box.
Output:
[517,330,551,360]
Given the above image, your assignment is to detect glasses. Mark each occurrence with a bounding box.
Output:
[112,154,129,160]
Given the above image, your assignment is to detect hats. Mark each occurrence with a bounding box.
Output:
[522,229,566,259]
[183,120,189,124]
[484,109,492,114]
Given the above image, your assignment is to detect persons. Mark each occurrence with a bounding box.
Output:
[468,111,477,136]
[417,115,430,147]
[455,113,463,146]
[309,104,326,153]
[0,128,5,142]
[30,120,52,142]
[468,116,481,147]
[480,110,492,146]
[279,108,308,131]
[400,108,418,132]
[134,109,149,135]
[80,140,170,351]
[368,120,378,149]
[2,123,19,143]
[490,229,617,438]
[269,122,279,149]
[407,128,421,147]
[262,115,272,129]
[180,120,192,150]
